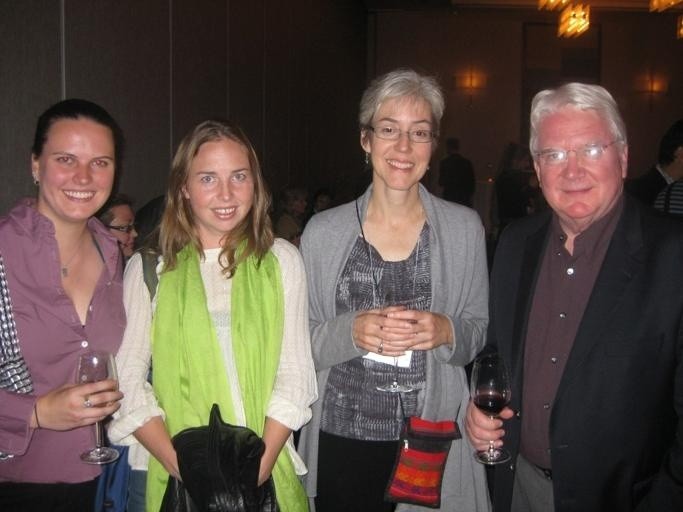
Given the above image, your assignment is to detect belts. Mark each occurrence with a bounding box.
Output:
[535,464,552,480]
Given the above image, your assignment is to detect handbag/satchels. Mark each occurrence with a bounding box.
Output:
[382,413,461,508]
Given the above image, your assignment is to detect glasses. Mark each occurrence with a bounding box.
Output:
[533,140,617,165]
[107,225,132,233]
[367,125,436,143]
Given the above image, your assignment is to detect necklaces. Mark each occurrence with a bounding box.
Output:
[363,206,423,394]
[60,251,78,279]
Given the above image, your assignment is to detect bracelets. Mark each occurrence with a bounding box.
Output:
[34,404,40,430]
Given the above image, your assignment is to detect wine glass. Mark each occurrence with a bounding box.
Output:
[377,292,425,393]
[74,351,120,464]
[471,356,511,464]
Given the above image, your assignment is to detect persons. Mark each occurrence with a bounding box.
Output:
[265,182,337,246]
[464,82,682,512]
[624,120,683,215]
[96,195,139,264]
[106,119,319,512]
[496,145,539,227]
[299,69,492,512]
[0,98,126,511]
[438,138,476,209]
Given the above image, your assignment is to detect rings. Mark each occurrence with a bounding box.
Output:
[83,394,92,410]
[378,339,383,354]
